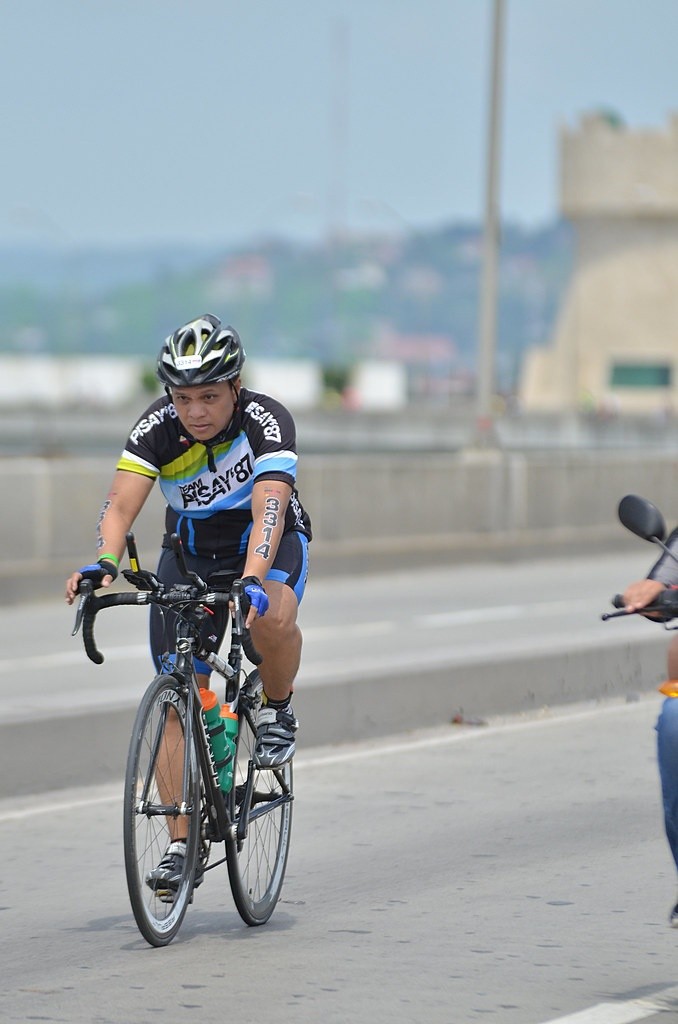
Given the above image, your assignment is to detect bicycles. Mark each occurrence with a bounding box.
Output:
[66,529,300,948]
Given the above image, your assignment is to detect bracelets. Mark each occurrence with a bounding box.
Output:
[97,553,119,567]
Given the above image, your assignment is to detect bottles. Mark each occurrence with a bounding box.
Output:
[200,688,229,761]
[215,704,238,794]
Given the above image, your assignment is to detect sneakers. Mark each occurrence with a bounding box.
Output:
[252,703,300,770]
[144,841,205,890]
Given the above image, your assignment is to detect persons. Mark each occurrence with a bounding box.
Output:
[621,580,677,930]
[64,313,312,892]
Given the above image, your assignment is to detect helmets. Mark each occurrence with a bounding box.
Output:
[154,313,246,388]
